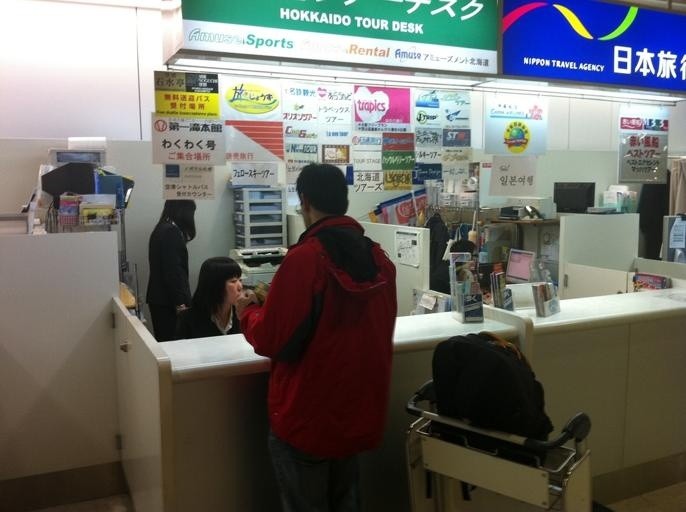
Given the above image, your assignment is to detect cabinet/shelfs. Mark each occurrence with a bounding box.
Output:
[232,185,288,250]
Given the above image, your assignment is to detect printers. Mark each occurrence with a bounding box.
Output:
[228,246,289,290]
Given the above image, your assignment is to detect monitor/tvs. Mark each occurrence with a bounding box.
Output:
[505,248,536,284]
[554,182,594,213]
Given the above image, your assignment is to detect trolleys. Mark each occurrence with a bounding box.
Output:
[406,379,592,512]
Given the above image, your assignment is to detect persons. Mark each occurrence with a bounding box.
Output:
[235,163,398,512]
[638,170,672,259]
[172,259,243,340]
[145,200,196,343]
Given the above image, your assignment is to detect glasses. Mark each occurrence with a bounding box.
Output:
[293,203,306,215]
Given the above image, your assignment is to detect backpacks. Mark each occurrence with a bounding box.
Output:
[433,330,554,466]
